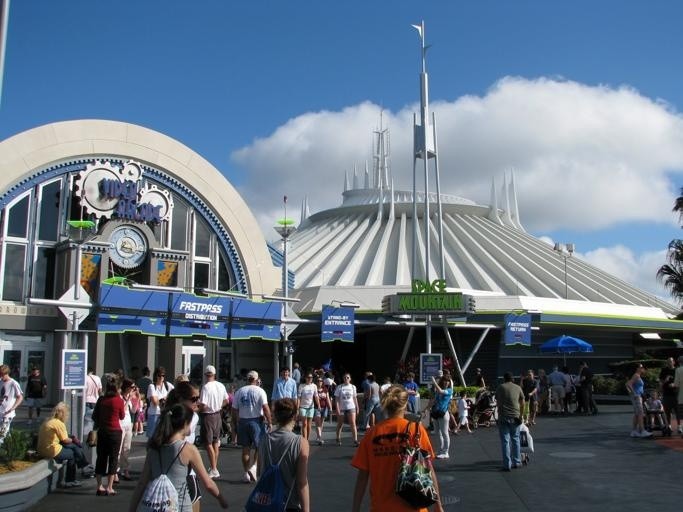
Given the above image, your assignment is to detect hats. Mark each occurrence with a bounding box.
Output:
[247,371,259,380]
[204,365,216,375]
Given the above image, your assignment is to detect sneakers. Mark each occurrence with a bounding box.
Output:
[630,431,639,437]
[83,464,95,475]
[208,468,221,479]
[668,424,682,434]
[437,452,449,459]
[133,432,145,436]
[449,428,475,435]
[96,477,132,495]
[315,439,359,447]
[539,407,598,415]
[640,432,653,437]
[248,464,258,481]
[506,464,522,470]
[242,473,251,483]
[525,420,536,426]
[65,480,82,487]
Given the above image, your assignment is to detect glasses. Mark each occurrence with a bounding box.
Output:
[186,396,200,402]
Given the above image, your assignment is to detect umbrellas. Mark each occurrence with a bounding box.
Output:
[540,334,595,370]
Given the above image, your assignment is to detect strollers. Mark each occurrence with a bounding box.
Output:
[639,387,675,439]
[466,389,496,430]
[194,391,234,446]
[517,418,530,465]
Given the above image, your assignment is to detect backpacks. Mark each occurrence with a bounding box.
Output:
[139,474,184,512]
[245,452,294,512]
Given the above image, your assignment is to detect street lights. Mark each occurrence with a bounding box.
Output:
[256,195,298,378]
[552,240,575,300]
[17,190,106,447]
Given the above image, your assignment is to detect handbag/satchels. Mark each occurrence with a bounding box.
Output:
[394,420,436,506]
[86,430,98,447]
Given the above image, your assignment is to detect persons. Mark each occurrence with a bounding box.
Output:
[126,405,227,511]
[1,360,483,497]
[346,384,445,512]
[520,369,538,425]
[494,372,526,471]
[250,397,312,511]
[536,363,599,416]
[623,356,683,438]
